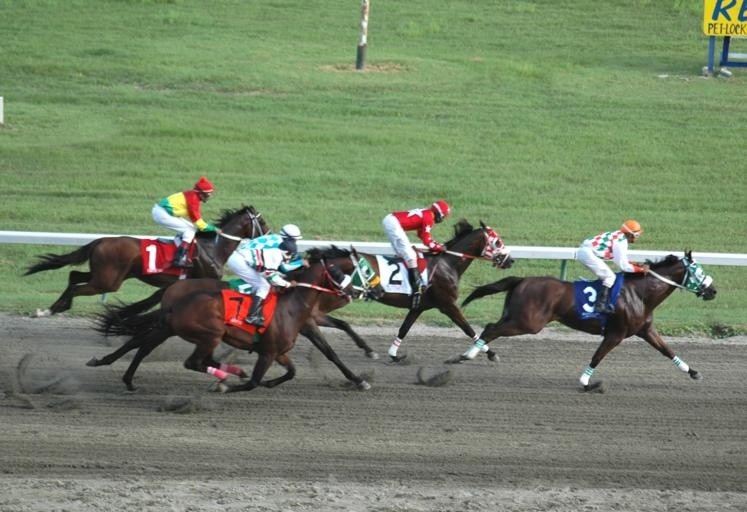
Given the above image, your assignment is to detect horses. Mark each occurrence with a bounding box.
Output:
[89,246,386,396]
[21,206,270,317]
[460,247,718,392]
[360,219,516,363]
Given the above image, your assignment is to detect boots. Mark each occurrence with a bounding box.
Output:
[245,296,265,326]
[172,246,193,268]
[409,267,432,292]
[595,285,615,314]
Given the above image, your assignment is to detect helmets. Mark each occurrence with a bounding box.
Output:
[432,200,448,219]
[621,220,641,238]
[280,224,302,240]
[193,178,213,193]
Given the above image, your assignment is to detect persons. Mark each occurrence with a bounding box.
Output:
[151,176,222,270]
[236,222,310,273]
[575,219,651,315]
[226,239,303,328]
[381,200,451,296]
